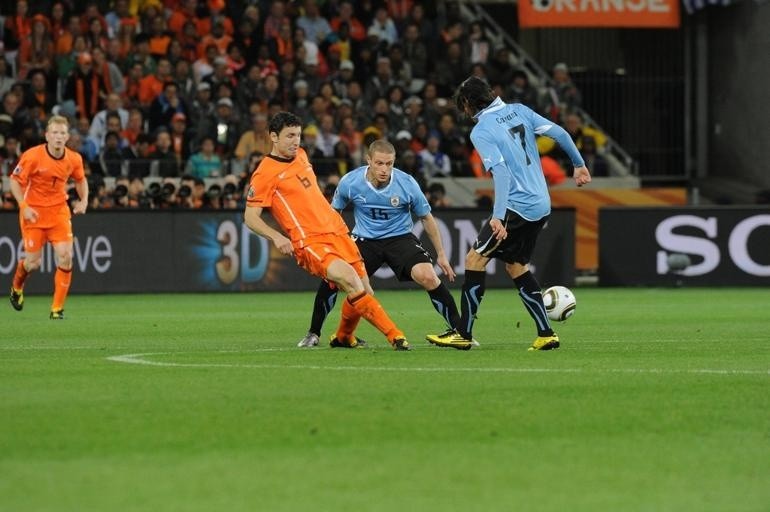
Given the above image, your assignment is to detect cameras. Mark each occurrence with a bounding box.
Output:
[138,182,160,199]
[179,186,191,197]
[224,183,236,194]
[162,183,175,195]
[325,183,337,196]
[114,185,127,197]
[203,185,221,202]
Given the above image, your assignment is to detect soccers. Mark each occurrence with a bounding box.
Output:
[542,286,575,321]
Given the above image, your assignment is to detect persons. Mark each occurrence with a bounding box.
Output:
[425,76,593,350]
[1,2,611,212]
[298,139,482,348]
[245,112,412,350]
[9,115,89,319]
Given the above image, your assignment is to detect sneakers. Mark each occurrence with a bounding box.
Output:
[9,286,24,311]
[527,335,560,352]
[50,309,64,320]
[297,332,319,348]
[329,333,368,348]
[393,336,409,349]
[426,328,472,350]
